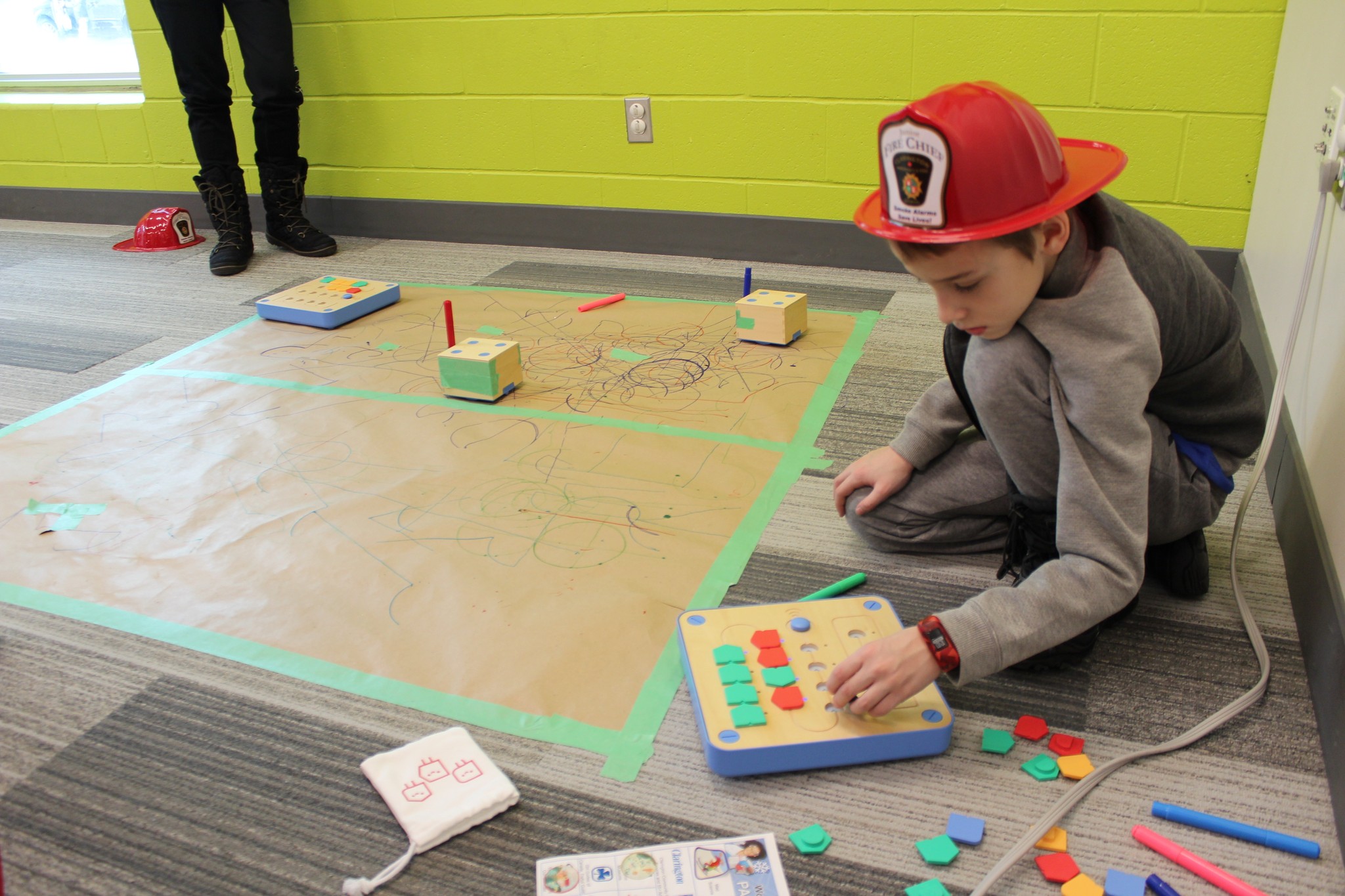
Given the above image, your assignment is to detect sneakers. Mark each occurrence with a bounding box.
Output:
[1141,529,1210,600]
[977,502,1140,676]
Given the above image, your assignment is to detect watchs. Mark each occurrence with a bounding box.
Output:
[919,616,959,673]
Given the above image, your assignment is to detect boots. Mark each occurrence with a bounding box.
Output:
[194,167,254,275]
[254,150,336,257]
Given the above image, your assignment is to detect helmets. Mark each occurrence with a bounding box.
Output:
[113,207,206,253]
[853,81,1128,242]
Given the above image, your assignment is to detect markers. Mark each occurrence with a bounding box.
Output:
[444,300,455,349]
[1143,872,1176,894]
[743,266,751,296]
[799,573,867,602]
[578,293,627,313]
[1151,801,1322,860]
[1133,824,1264,895]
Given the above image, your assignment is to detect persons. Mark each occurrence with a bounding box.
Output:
[150,0,338,276]
[828,82,1265,716]
